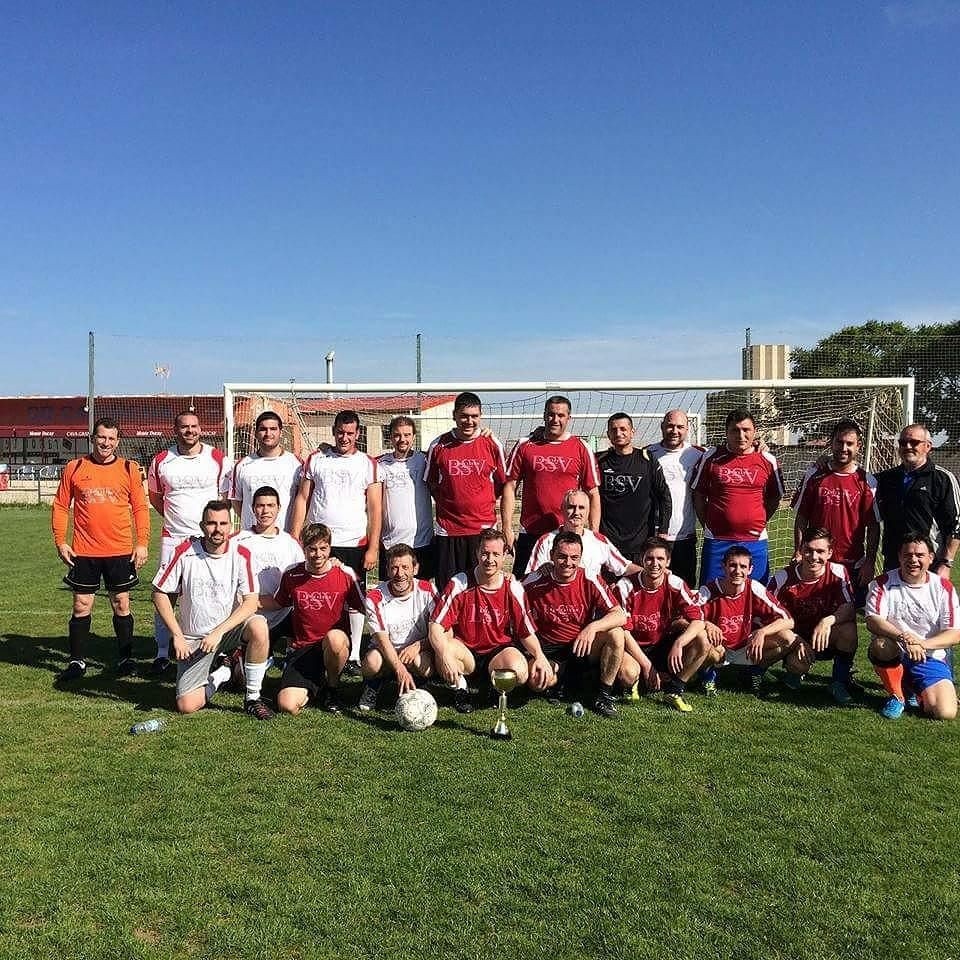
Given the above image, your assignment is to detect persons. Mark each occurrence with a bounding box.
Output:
[50,418,151,681]
[148,412,960,720]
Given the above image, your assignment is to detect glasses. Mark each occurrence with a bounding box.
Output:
[898,439,927,448]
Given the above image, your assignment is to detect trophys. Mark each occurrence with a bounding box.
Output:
[488,668,519,740]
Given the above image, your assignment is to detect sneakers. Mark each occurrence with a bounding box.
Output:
[879,693,907,720]
[550,688,570,703]
[592,695,617,715]
[223,649,245,691]
[662,687,692,712]
[905,693,921,711]
[243,697,274,720]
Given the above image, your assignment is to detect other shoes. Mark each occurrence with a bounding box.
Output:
[831,680,865,705]
[358,675,379,710]
[747,673,764,693]
[68,654,87,674]
[319,690,342,713]
[487,684,520,704]
[698,670,720,701]
[153,657,172,673]
[343,660,363,676]
[784,672,803,695]
[622,680,640,702]
[455,688,478,713]
[120,656,138,676]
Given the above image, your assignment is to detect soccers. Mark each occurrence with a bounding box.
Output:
[395,688,438,732]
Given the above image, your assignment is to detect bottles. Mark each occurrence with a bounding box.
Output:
[572,702,584,717]
[130,717,164,733]
